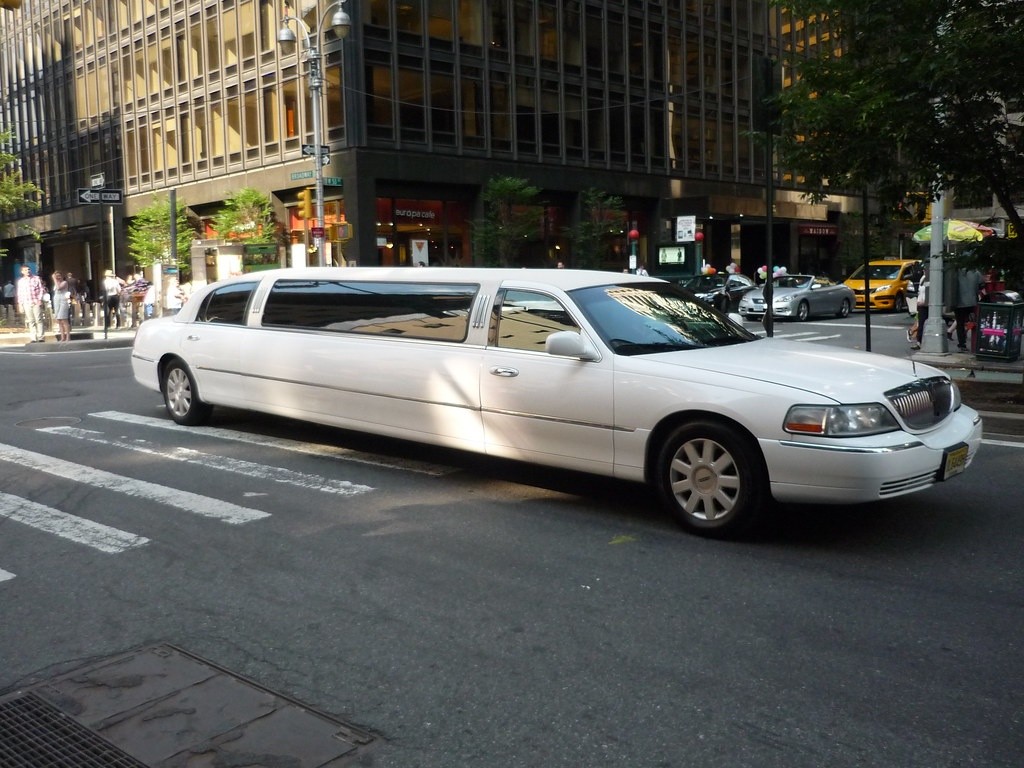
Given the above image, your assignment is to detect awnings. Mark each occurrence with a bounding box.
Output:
[798,223,838,235]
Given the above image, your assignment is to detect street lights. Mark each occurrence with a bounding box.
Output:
[312,0,353,266]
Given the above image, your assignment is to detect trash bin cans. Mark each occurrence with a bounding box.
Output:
[972,290,1024,363]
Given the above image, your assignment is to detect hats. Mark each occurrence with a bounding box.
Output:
[104,270,114,276]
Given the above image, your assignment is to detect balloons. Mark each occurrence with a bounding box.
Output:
[726,263,740,274]
[700,264,716,275]
[757,265,787,279]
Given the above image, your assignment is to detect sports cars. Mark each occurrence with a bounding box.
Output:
[738,274,856,323]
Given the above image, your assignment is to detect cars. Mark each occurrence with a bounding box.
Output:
[679,272,758,315]
[842,259,924,314]
[128,266,985,544]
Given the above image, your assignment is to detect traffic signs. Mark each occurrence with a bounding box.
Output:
[77,187,123,205]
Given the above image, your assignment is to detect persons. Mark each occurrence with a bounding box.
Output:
[0,265,152,343]
[712,286,731,312]
[418,260,427,268]
[558,262,565,269]
[660,247,684,263]
[622,263,649,278]
[906,256,1003,353]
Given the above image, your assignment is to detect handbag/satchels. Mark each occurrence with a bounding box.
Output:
[917,275,926,307]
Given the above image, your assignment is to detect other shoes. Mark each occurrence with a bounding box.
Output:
[910,344,921,350]
[906,329,913,342]
[957,347,971,353]
[946,330,953,340]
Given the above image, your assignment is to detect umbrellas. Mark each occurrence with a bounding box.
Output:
[910,219,995,252]
